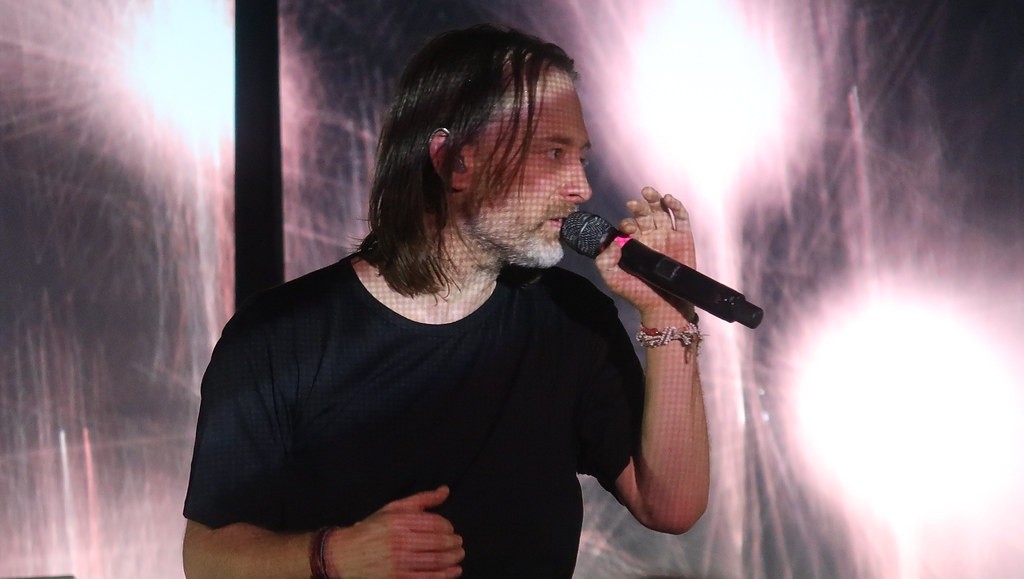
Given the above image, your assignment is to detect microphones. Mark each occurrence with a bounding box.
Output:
[560,211,764,330]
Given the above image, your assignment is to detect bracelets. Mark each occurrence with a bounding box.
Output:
[636,312,702,348]
[309,526,339,579]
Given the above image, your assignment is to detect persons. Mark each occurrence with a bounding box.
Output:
[182,27,710,579]
